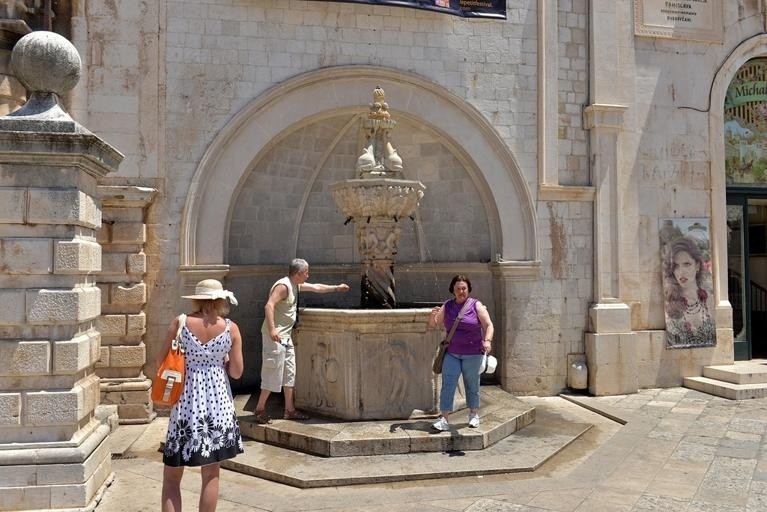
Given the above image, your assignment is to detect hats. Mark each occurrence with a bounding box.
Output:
[478,350,497,376]
[180,279,239,306]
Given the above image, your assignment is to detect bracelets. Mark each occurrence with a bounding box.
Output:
[483,338,492,343]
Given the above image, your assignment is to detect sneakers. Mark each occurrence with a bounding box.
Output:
[430,417,449,431]
[468,413,479,428]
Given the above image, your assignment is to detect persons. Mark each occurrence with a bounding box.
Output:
[157,275,244,512]
[250,258,349,424]
[664,233,713,346]
[427,273,494,432]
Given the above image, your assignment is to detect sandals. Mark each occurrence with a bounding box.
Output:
[253,407,273,424]
[283,410,309,420]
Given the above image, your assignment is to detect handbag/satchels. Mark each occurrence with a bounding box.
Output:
[433,339,450,374]
[151,340,185,407]
